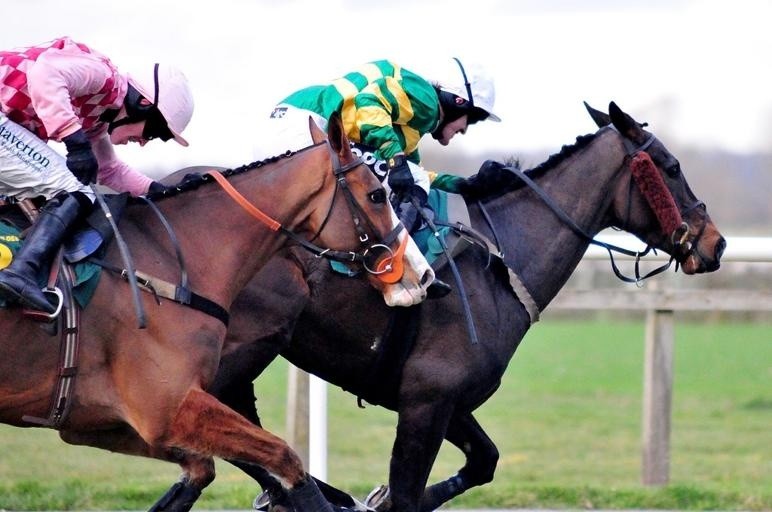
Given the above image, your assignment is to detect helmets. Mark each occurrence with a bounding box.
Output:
[431,56,503,122]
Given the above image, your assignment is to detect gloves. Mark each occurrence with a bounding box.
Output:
[61,127,98,184]
[386,154,415,204]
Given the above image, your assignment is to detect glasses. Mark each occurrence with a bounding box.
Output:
[452,55,482,125]
[142,108,159,140]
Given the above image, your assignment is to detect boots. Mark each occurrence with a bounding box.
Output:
[0,190,86,322]
[391,184,452,298]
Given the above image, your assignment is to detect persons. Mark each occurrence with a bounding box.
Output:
[267,55,502,303]
[1,36,207,318]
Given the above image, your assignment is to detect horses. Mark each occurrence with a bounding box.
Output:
[0,108,441,512]
[154,100,729,511]
